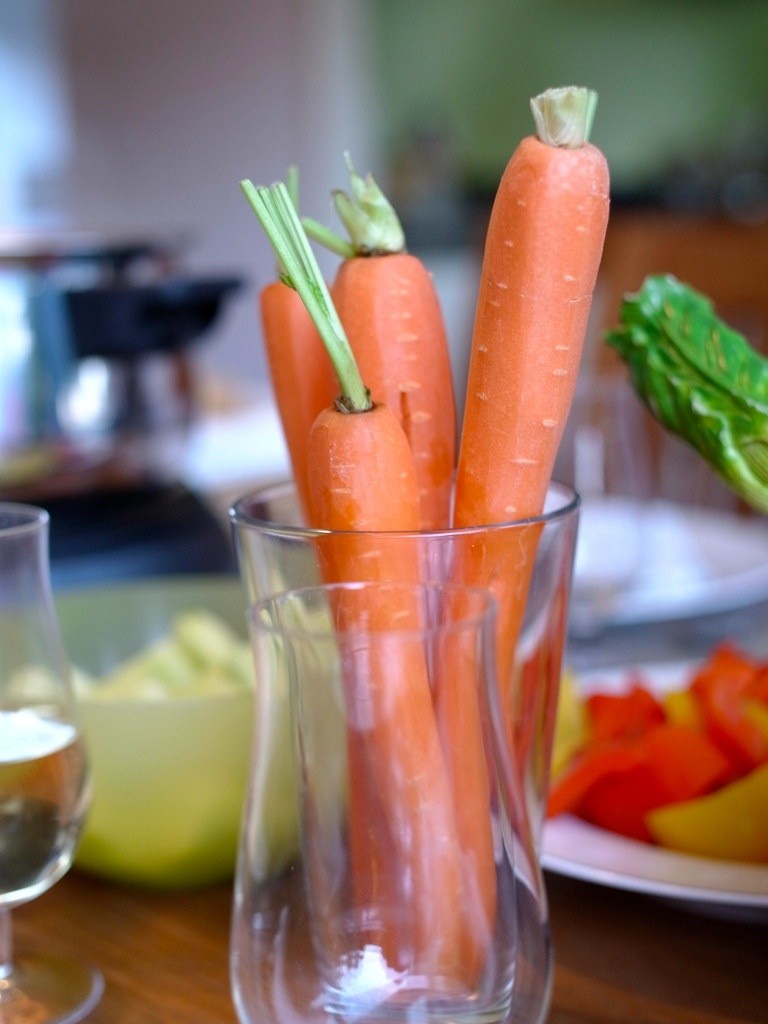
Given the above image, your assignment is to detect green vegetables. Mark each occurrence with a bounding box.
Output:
[602,271,768,517]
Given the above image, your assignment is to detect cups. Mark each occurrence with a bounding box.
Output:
[230,581,555,1024]
[228,479,582,870]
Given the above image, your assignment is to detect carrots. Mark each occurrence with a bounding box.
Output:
[235,85,609,1003]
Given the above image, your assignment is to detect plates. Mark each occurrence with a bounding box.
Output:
[566,497,768,657]
[539,658,768,905]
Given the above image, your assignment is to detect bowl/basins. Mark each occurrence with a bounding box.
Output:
[5,579,333,890]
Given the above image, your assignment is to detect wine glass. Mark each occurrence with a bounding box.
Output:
[551,378,653,671]
[0,502,106,1024]
